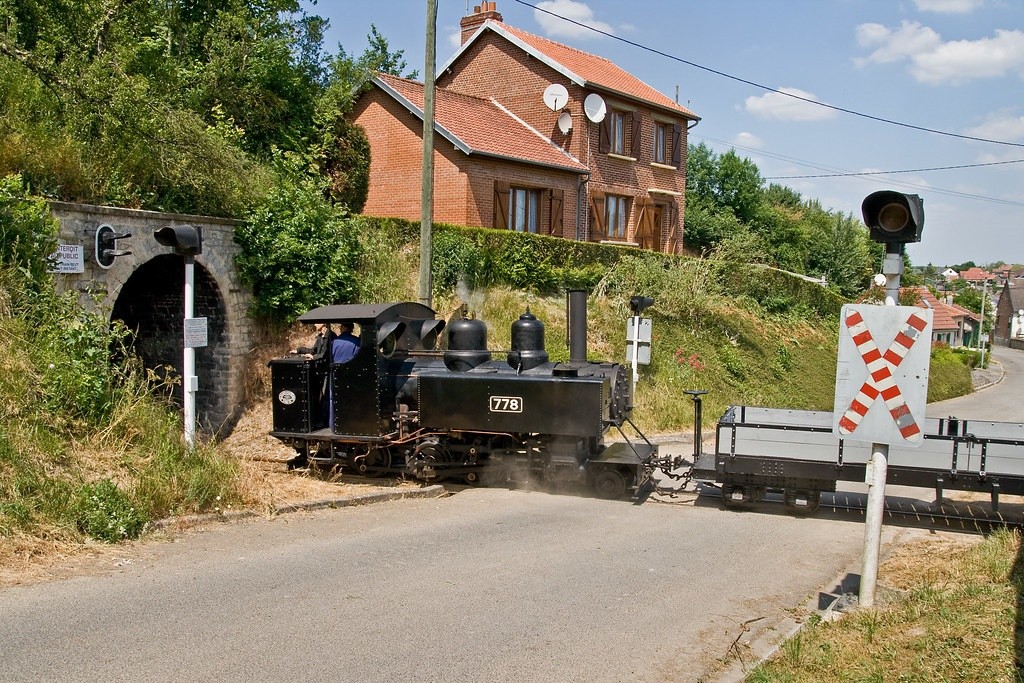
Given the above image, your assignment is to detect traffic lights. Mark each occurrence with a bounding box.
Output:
[628,294,654,316]
[153,222,203,256]
[861,190,922,243]
[94,222,134,269]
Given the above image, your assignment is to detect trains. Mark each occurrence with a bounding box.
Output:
[265,285,1024,519]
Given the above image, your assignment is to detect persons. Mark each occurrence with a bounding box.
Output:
[327,322,360,433]
[289,322,338,426]
